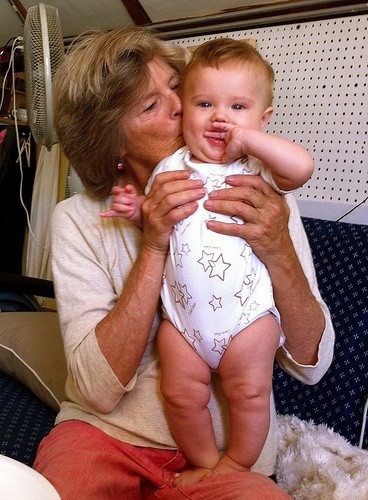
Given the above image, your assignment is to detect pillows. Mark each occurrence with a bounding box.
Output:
[0,311,68,412]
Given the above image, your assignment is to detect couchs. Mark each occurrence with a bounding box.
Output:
[0,216,368,500]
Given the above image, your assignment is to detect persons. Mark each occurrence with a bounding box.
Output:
[100,39,314,487]
[32,28,335,500]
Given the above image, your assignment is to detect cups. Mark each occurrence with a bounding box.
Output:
[11,108,28,124]
